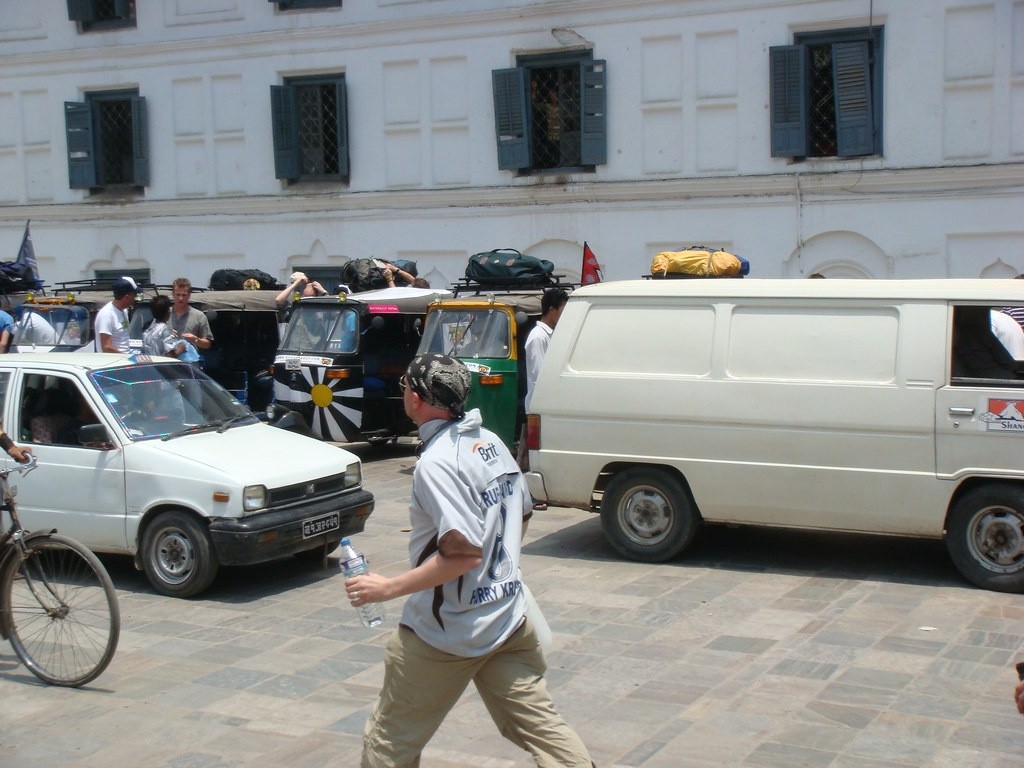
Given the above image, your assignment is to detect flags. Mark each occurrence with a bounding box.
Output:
[17,224,46,297]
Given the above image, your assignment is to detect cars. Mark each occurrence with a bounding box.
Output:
[0,352,378,601]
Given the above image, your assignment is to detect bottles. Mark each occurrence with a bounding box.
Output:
[338,538,386,628]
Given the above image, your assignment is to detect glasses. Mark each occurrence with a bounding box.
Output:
[398,374,412,393]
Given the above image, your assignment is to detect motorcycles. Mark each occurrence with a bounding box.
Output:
[0,274,458,451]
[408,285,574,464]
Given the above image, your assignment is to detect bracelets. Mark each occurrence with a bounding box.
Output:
[385,275,395,282]
[321,292,327,296]
[395,268,402,274]
[193,337,199,345]
[0,431,15,454]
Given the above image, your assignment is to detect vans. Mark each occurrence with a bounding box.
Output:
[520,274,1023,595]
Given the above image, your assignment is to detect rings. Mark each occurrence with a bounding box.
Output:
[355,592,357,597]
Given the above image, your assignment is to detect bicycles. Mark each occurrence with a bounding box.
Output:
[0,452,122,689]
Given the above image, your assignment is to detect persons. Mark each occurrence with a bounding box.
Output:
[532,80,571,168]
[166,278,214,415]
[0,309,65,354]
[275,271,356,351]
[990,274,1024,361]
[808,274,825,279]
[525,290,569,510]
[0,423,32,640]
[344,353,596,768]
[94,277,144,354]
[1015,662,1024,715]
[383,263,431,289]
[141,295,186,423]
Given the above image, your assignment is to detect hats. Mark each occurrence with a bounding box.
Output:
[112,277,144,294]
[290,272,309,285]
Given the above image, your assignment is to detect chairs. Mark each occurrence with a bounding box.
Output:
[31,414,60,443]
[457,318,508,359]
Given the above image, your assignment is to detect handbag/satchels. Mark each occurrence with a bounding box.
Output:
[466,248,554,285]
[0,261,36,293]
[207,268,277,290]
[342,258,396,289]
